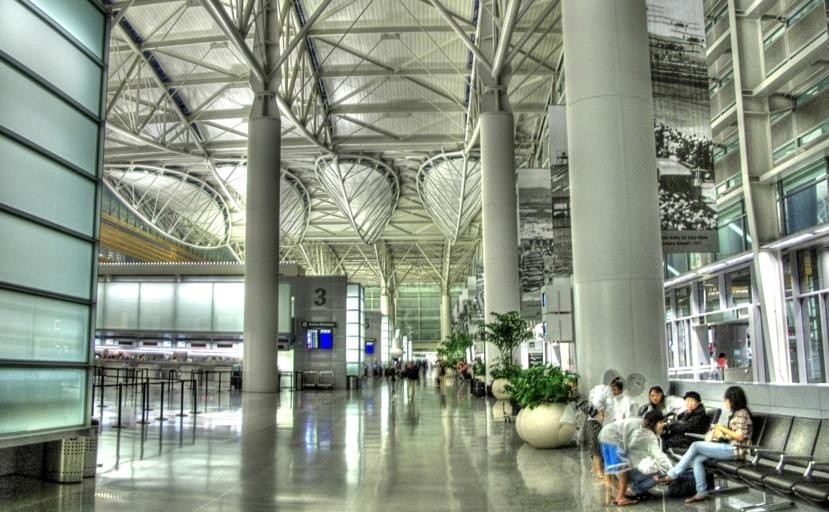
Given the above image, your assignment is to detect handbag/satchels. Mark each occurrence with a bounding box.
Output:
[666,467,716,499]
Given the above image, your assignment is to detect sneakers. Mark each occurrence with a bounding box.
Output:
[589,470,706,506]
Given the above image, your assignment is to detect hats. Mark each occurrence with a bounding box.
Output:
[682,391,702,401]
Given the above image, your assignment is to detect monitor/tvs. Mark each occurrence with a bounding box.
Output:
[365,341,374,354]
[306,329,333,349]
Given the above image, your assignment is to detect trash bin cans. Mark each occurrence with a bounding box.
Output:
[347,375,358,390]
[42,419,99,484]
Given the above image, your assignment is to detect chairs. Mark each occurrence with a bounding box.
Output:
[657,404,829,512]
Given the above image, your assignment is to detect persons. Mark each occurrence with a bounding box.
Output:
[374,357,469,387]
[709,342,713,356]
[718,353,728,367]
[589,369,753,504]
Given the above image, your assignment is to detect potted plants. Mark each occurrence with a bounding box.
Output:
[478,310,530,400]
[508,363,582,448]
[473,357,485,383]
[437,334,458,376]
